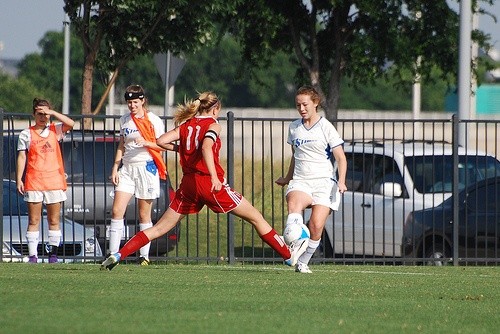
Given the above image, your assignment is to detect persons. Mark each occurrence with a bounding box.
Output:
[103,84,167,265]
[17,98,75,264]
[276,88,348,273]
[101,93,307,271]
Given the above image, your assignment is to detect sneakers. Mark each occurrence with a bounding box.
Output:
[294,262,312,273]
[138,257,149,266]
[284,238,308,267]
[49,255,58,263]
[27,254,38,263]
[100,253,120,271]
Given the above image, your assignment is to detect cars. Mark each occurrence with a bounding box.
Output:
[401,177,499,265]
[2,179,103,264]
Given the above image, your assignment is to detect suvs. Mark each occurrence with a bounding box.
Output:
[303,137,499,264]
[2,133,180,255]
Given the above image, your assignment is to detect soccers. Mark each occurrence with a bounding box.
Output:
[283,223,311,246]
